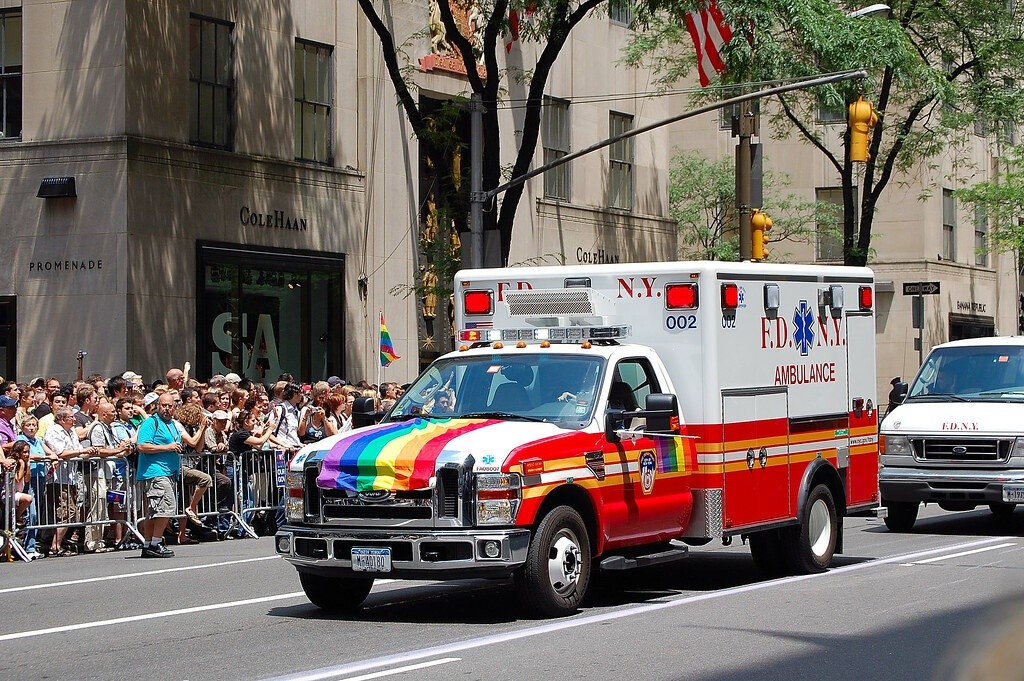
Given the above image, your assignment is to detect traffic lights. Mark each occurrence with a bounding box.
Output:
[752,212,773,262]
[848,97,878,163]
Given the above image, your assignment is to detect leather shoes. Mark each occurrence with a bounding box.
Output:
[146,542,174,558]
[141,546,155,557]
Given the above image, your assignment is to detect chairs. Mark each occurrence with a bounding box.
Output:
[612,381,643,427]
[485,383,532,411]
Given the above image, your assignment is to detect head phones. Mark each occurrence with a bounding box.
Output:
[285,385,295,400]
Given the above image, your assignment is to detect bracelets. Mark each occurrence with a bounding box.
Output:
[77,367,82,370]
[79,449,83,454]
[443,386,448,390]
[322,416,327,422]
[302,417,307,422]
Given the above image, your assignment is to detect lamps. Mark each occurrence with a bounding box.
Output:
[36,177,77,199]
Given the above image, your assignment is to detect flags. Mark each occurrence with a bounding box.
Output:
[503,0,521,54]
[380,315,401,367]
[684,0,755,88]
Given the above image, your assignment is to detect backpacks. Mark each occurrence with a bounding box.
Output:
[77,423,109,475]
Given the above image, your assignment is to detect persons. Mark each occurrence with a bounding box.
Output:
[420,122,461,338]
[557,383,625,430]
[0,362,456,563]
[927,371,956,395]
[888,377,903,413]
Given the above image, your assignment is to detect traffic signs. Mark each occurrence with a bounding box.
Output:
[903,281,941,295]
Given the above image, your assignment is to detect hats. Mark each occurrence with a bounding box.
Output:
[0,395,18,407]
[151,379,163,389]
[212,410,230,421]
[122,372,142,379]
[29,377,45,386]
[142,392,159,409]
[328,376,345,386]
[302,385,311,391]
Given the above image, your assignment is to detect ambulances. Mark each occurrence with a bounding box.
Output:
[270,261,884,617]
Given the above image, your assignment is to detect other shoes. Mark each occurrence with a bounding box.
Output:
[3,512,140,561]
[172,504,278,543]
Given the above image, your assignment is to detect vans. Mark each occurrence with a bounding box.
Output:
[875,335,1024,531]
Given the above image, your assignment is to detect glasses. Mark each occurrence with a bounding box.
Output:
[66,414,74,419]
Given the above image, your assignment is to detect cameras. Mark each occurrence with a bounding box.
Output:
[311,407,319,412]
[79,351,88,358]
[177,447,186,454]
